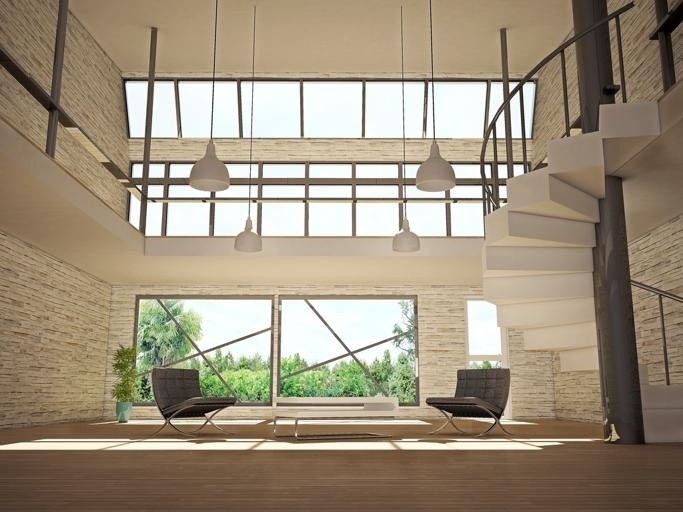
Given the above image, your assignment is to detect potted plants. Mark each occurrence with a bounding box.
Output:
[109,340,138,422]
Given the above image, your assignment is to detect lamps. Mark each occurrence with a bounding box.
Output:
[389,4,424,253]
[232,5,264,254]
[186,0,234,193]
[413,0,457,194]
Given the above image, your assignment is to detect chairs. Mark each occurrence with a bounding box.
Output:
[145,366,239,439]
[426,367,517,439]
